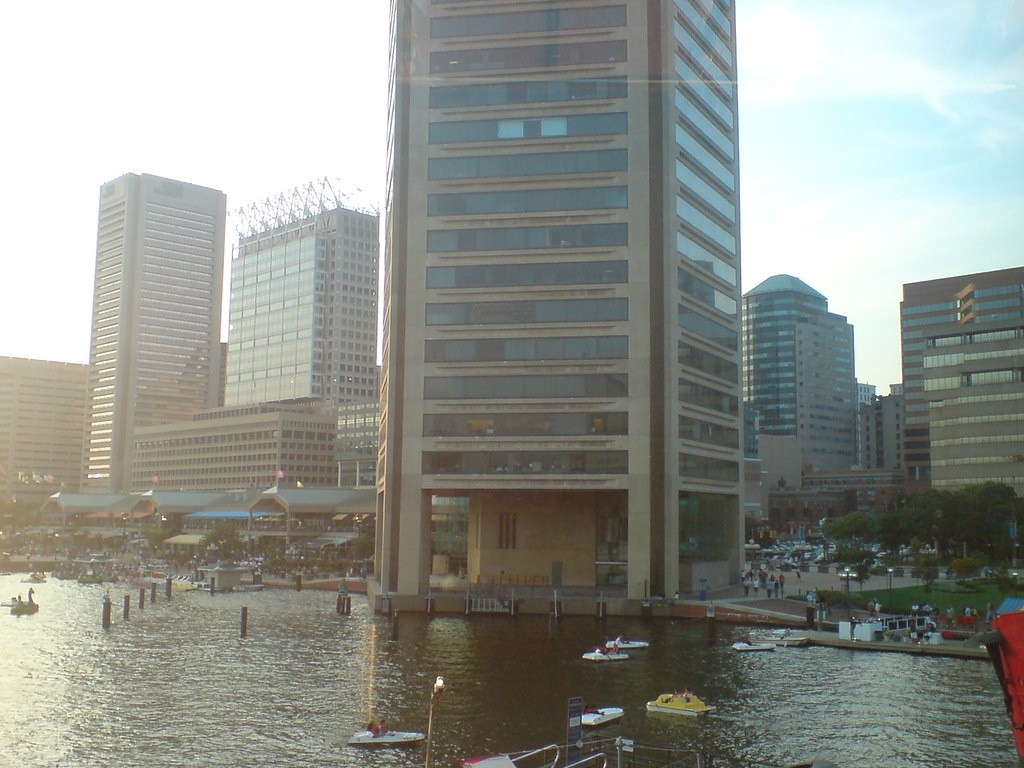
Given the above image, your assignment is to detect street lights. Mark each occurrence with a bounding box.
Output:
[886,565,894,617]
[836,568,859,623]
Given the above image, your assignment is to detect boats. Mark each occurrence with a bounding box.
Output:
[645,688,719,717]
[0,551,199,614]
[605,640,650,649]
[580,706,625,727]
[962,608,1024,768]
[582,652,630,661]
[731,639,777,651]
[347,726,427,744]
[748,627,811,647]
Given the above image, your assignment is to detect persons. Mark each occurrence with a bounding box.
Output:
[867,598,994,645]
[699,567,832,616]
